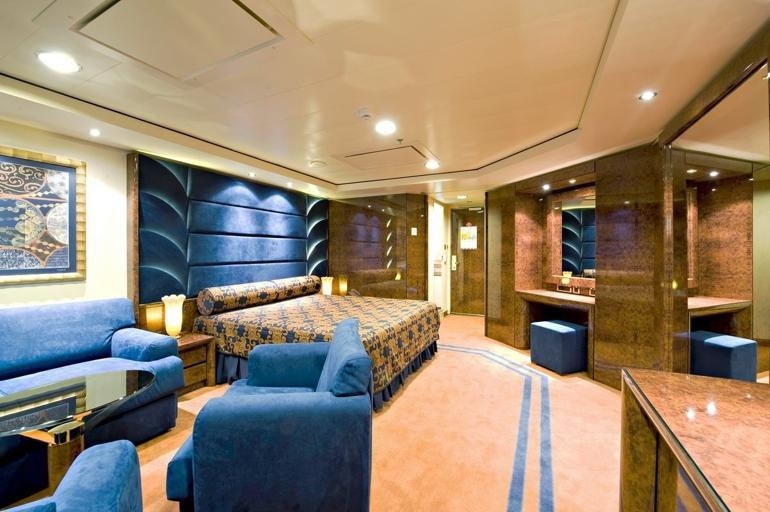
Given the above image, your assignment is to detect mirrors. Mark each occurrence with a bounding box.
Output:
[561,206,595,278]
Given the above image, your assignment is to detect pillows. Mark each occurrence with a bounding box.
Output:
[197,280,278,315]
[350,268,396,284]
[274,274,323,299]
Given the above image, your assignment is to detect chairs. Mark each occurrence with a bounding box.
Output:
[1,440,152,512]
[162,313,374,512]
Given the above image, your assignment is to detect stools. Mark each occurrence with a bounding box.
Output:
[691,331,761,384]
[528,320,588,378]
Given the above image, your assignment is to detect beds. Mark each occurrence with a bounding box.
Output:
[194,292,443,415]
[350,268,407,299]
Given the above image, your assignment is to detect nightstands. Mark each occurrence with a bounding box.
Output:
[160,328,217,390]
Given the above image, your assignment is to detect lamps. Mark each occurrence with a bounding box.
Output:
[318,276,334,293]
[162,292,188,338]
[337,273,349,295]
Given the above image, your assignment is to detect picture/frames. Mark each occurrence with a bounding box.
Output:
[0,141,91,290]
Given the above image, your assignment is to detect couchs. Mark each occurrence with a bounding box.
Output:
[1,291,186,399]
